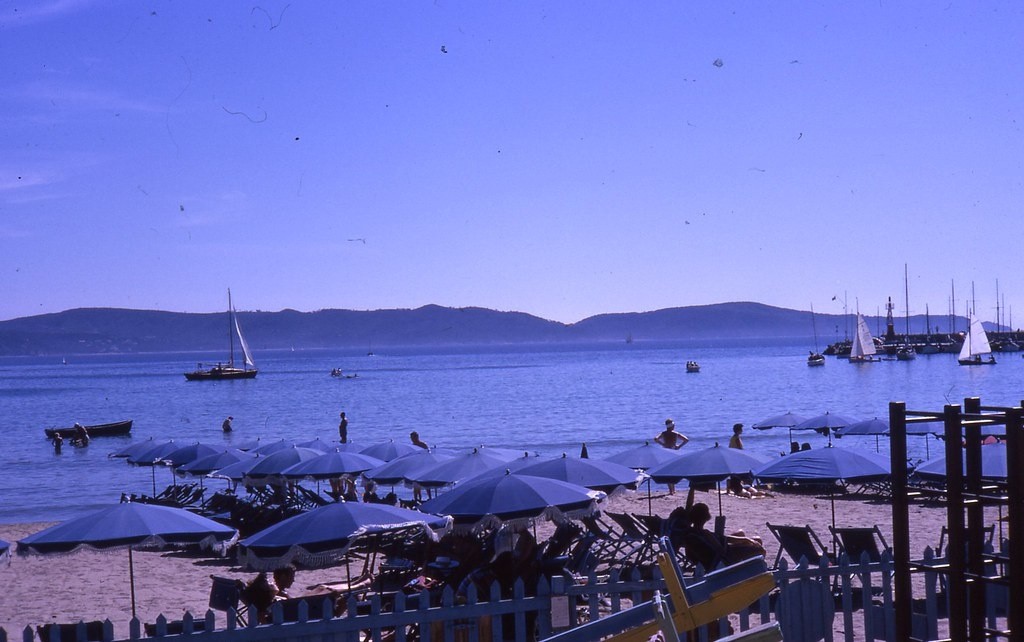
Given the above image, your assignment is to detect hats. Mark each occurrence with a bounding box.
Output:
[74,423,79,427]
[688,503,711,521]
[666,419,675,428]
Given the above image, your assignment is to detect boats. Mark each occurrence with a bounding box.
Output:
[44,419,134,438]
[687,366,700,372]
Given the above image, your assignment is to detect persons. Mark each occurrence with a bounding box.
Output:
[669,506,692,526]
[802,443,811,451]
[74,423,90,442]
[268,565,295,603]
[727,477,752,497]
[691,503,762,548]
[655,419,688,495]
[247,569,377,624]
[410,431,431,501]
[512,522,537,552]
[222,416,233,431]
[741,483,774,497]
[52,433,63,449]
[339,412,348,442]
[729,424,744,495]
[793,441,800,452]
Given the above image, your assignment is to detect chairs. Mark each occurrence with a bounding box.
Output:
[120,469,1011,627]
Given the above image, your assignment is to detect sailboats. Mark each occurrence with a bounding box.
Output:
[896,264,916,360]
[850,297,881,362]
[184,286,258,380]
[922,304,939,354]
[807,303,825,365]
[958,308,997,364]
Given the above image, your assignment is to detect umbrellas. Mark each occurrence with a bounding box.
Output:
[1,411,1007,618]
